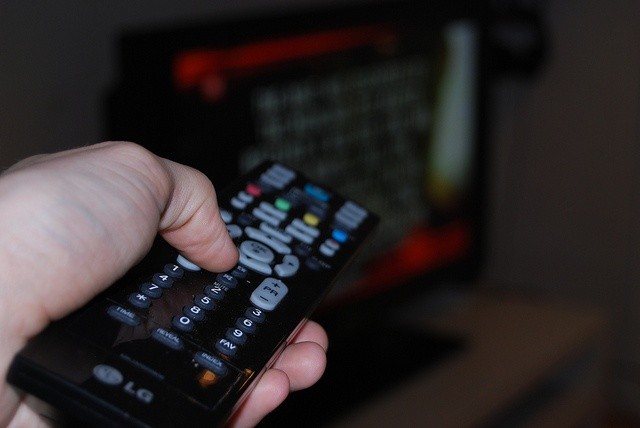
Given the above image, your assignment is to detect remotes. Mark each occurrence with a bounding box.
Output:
[7,156,380,428]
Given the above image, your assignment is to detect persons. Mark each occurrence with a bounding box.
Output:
[0,139,329,428]
